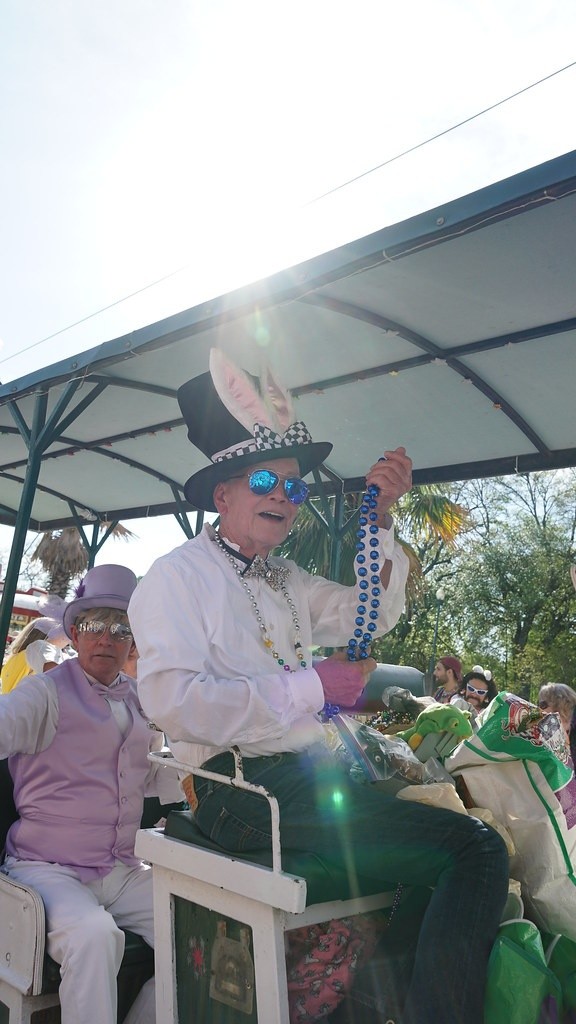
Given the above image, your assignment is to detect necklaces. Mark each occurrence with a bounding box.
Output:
[441,689,454,697]
[320,457,386,721]
[216,534,307,673]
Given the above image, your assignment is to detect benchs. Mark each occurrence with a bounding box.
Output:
[0,864,63,1024]
[132,716,397,1024]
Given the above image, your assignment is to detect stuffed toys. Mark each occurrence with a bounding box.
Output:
[397,704,473,750]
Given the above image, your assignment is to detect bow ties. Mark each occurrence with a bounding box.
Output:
[219,540,292,592]
[91,681,130,702]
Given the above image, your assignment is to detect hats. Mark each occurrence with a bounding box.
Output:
[63,564,138,640]
[441,656,463,681]
[176,348,333,512]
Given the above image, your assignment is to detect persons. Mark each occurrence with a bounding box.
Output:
[538,683,576,771]
[126,441,511,1024]
[451,668,497,714]
[432,656,463,702]
[0,564,190,1024]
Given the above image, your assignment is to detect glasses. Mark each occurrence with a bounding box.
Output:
[467,684,488,695]
[536,700,553,710]
[224,469,309,505]
[76,621,133,643]
